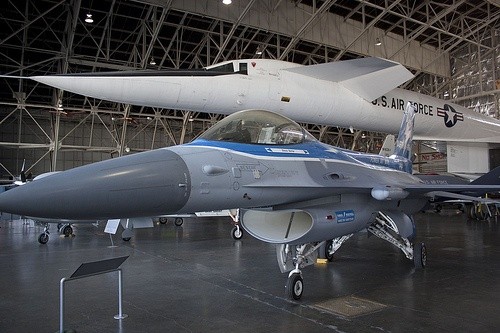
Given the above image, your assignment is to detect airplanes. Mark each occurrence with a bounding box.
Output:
[1,54,497,145]
[0,101,500,301]
